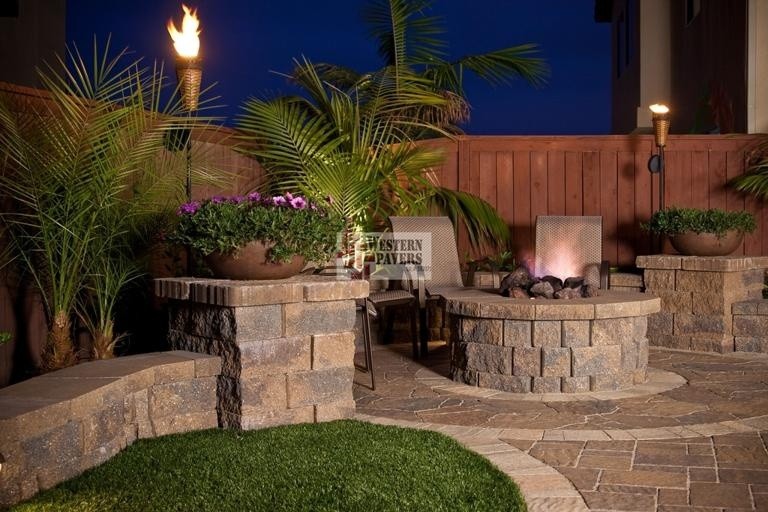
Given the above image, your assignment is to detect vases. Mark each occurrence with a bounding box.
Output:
[206,232,311,283]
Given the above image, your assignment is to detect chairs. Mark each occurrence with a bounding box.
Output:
[529,213,610,290]
[324,256,420,373]
[387,213,480,364]
[312,260,378,394]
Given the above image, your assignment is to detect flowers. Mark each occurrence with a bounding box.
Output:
[157,185,354,270]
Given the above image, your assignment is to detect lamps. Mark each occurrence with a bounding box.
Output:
[163,4,206,201]
[647,102,673,214]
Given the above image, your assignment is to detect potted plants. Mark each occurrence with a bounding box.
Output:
[640,209,679,256]
[664,203,760,258]
